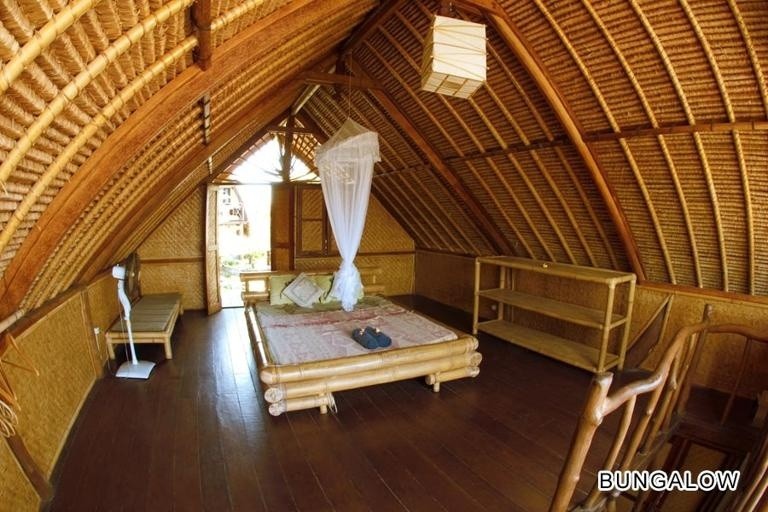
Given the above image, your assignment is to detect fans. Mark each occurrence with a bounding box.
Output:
[111,252,158,380]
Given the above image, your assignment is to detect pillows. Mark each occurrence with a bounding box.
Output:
[267,273,338,306]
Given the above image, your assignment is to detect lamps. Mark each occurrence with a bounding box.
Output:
[419,0,489,101]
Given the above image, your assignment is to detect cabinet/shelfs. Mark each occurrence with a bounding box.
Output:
[472,254,636,385]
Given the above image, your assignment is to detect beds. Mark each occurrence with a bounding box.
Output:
[105,294,184,360]
[239,268,483,419]
